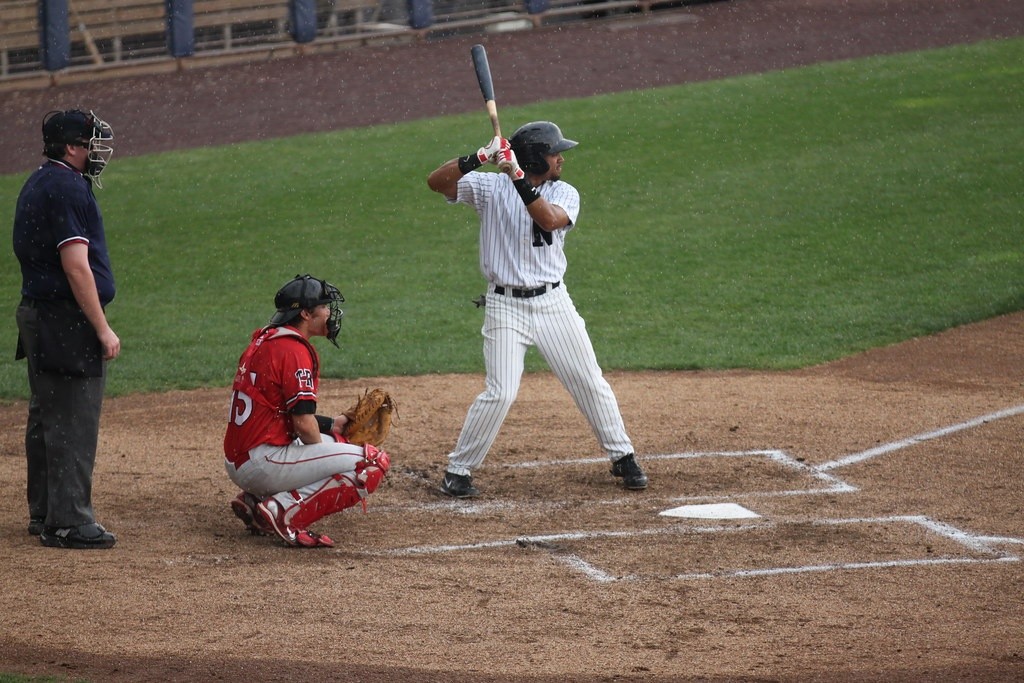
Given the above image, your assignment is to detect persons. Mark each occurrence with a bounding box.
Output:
[223,276,390,546]
[428,121,648,501]
[10,108,120,548]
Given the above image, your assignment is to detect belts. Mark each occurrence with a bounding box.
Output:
[19,296,40,309]
[494,281,561,299]
[234,452,251,471]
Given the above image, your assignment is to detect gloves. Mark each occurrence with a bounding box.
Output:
[477,136,525,181]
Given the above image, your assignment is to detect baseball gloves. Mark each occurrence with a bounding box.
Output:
[342,388,395,447]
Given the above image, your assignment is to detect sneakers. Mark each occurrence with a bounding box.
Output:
[28,516,116,550]
[440,472,480,499]
[231,492,300,545]
[612,453,647,489]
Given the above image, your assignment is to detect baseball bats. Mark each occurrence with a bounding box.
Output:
[469,43,511,174]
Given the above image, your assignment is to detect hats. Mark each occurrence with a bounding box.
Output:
[43,111,110,144]
[270,275,333,324]
[508,121,579,175]
